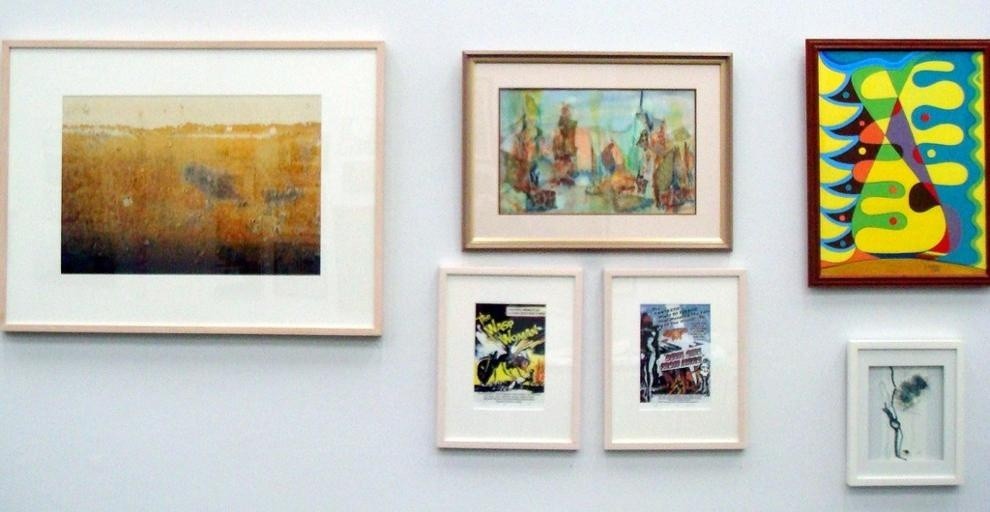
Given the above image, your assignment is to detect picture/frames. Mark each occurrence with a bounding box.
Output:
[2,38,388,337]
